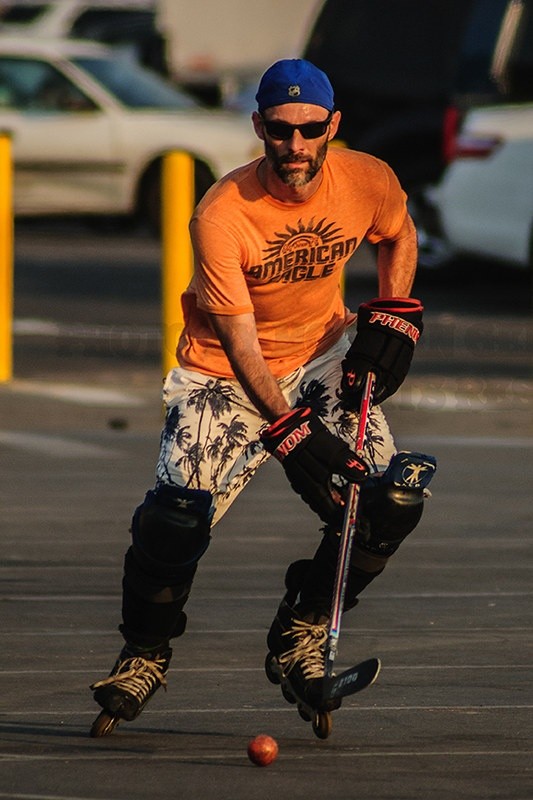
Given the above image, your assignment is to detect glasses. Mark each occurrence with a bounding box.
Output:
[259,107,335,140]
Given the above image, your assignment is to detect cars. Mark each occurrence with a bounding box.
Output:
[0,0,268,235]
[438,104,533,269]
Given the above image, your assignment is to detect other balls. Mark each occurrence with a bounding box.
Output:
[248,735,279,767]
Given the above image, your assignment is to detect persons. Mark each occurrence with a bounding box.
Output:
[89,59,436,740]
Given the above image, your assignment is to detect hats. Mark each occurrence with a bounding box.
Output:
[255,58,334,113]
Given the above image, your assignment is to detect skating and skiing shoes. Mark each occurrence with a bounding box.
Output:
[88,626,174,737]
[267,558,342,740]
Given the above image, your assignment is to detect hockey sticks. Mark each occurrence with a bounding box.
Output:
[321,372,381,701]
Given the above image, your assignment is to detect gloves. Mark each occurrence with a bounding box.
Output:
[341,297,425,409]
[260,406,370,541]
